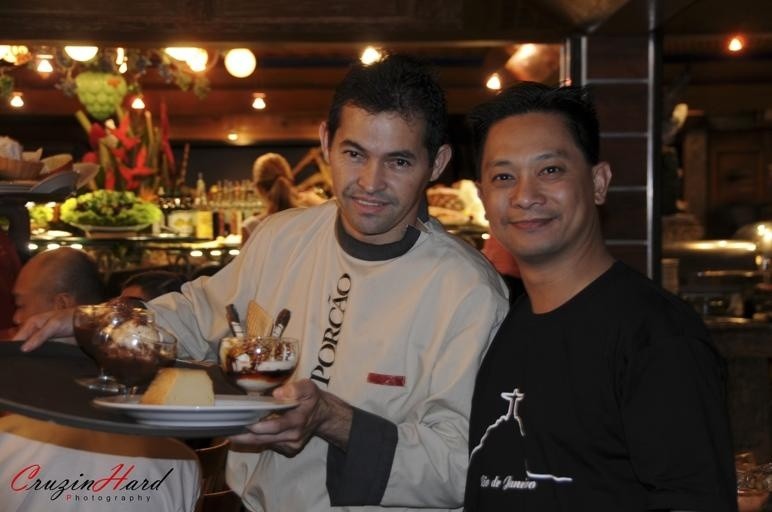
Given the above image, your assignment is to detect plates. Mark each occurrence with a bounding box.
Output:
[75,160,100,189]
[99,395,297,430]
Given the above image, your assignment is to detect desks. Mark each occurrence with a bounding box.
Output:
[148,240,240,279]
[663,238,757,315]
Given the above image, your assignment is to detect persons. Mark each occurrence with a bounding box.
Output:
[464,82,740,510]
[0,53,510,511]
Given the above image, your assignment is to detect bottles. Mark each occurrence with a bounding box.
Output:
[196,175,261,218]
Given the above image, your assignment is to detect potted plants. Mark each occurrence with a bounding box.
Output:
[57,190,162,237]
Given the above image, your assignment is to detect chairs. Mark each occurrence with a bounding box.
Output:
[661,213,705,242]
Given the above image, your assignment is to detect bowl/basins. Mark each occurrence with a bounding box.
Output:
[27,169,79,191]
[38,152,72,176]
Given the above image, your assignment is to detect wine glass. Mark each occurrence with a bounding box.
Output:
[76,304,182,400]
[219,335,299,395]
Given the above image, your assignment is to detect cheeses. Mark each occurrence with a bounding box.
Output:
[137,367,215,406]
